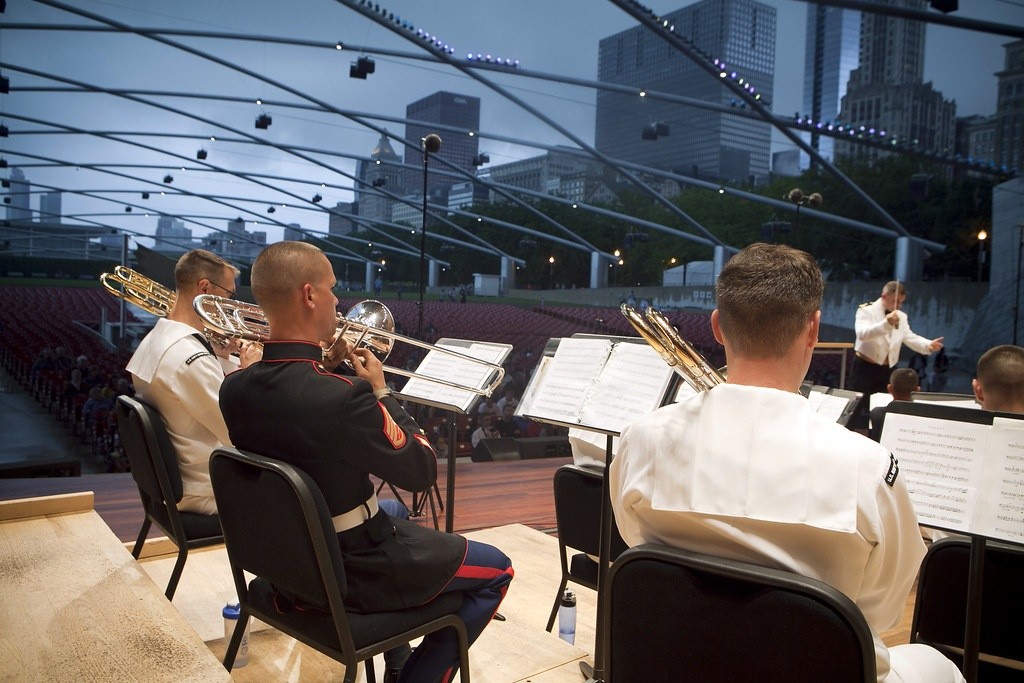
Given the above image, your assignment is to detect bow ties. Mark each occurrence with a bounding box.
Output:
[885,309,891,315]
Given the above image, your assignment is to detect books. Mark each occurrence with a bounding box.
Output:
[876,411,1024,545]
[523,336,676,434]
[399,344,511,411]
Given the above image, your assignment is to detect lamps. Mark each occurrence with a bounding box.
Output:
[100,50,672,252]
[0,0,12,244]
[931,0,960,14]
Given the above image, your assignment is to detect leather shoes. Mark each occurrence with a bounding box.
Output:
[384,667,403,683]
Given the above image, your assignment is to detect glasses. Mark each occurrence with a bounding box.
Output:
[210,282,237,300]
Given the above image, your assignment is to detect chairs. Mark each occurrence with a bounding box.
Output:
[0,284,1024,683]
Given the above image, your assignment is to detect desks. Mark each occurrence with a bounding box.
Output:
[0,491,237,683]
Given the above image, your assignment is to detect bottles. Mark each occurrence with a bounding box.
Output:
[560,591,577,645]
[222,599,250,668]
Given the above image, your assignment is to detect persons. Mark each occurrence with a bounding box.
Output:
[125,249,263,515]
[382,358,574,460]
[847,281,944,430]
[219,241,514,683]
[870,368,921,430]
[31,345,136,459]
[609,244,964,683]
[972,345,1024,414]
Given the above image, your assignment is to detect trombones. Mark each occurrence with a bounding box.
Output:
[193,293,506,397]
[620,302,726,393]
[99,265,244,354]
